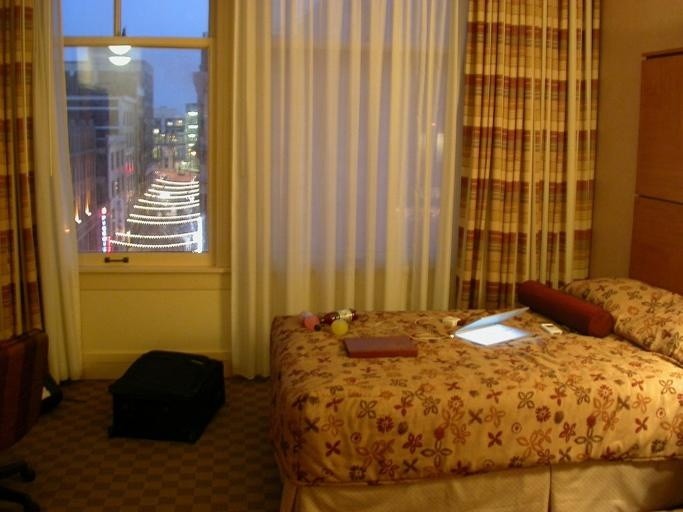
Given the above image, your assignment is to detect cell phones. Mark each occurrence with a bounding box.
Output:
[540,321,562,336]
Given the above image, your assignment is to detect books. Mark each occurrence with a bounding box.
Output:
[343,335,418,357]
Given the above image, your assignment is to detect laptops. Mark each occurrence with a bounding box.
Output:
[454,306,536,348]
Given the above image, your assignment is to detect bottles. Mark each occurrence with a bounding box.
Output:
[320,308,358,323]
[298,310,321,331]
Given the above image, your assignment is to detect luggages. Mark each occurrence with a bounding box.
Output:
[106,348,227,444]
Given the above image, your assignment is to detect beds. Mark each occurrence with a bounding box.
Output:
[270,277,683,512]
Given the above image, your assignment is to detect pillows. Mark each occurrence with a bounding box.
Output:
[518,280,612,337]
[563,278,683,369]
